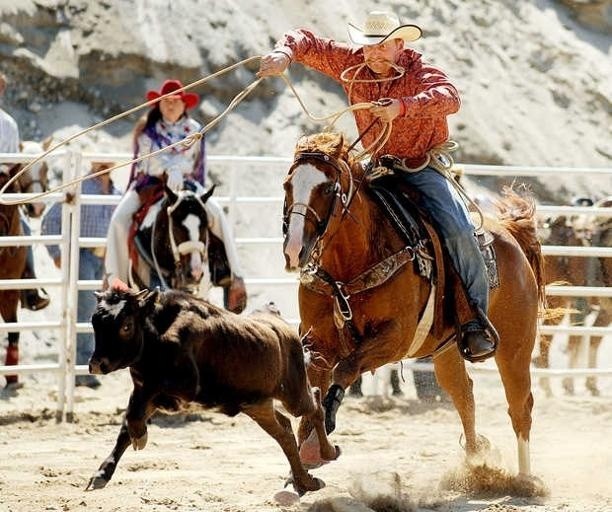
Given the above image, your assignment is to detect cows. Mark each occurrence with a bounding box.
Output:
[84,287,340,497]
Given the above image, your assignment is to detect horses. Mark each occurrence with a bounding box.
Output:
[0,163,28,390]
[540,200,611,397]
[135,184,217,301]
[19,136,54,217]
[283,133,581,494]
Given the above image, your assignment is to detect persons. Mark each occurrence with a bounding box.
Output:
[0,71,52,314]
[38,161,128,389]
[253,10,494,359]
[103,79,249,317]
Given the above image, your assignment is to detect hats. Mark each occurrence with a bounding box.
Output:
[147,80,199,112]
[345,11,424,47]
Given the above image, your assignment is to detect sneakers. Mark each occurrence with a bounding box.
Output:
[461,326,493,357]
